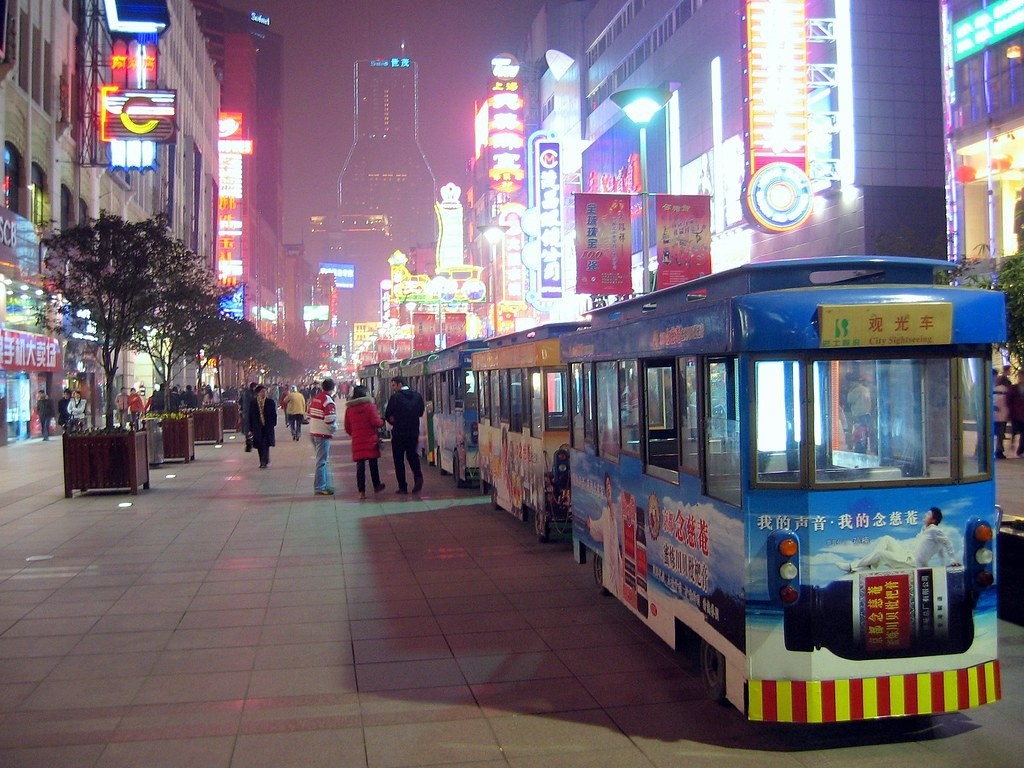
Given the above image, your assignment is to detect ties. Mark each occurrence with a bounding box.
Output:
[260,400,262,409]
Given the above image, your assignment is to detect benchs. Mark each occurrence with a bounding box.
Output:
[513,412,901,489]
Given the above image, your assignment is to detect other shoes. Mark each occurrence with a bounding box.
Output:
[412,485,422,494]
[293,436,295,441]
[395,489,408,494]
[836,561,855,572]
[297,437,299,441]
[259,463,267,468]
[996,453,1005,459]
[315,488,335,495]
[373,482,385,493]
[359,492,365,498]
[245,449,251,452]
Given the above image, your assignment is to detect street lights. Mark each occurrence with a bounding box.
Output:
[405,300,418,357]
[475,222,511,337]
[428,272,450,349]
[610,88,669,292]
[355,317,399,363]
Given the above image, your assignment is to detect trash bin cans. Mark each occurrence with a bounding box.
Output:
[141,418,164,469]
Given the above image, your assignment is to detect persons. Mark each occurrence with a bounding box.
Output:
[300,381,323,420]
[495,427,513,511]
[284,385,306,441]
[835,506,961,572]
[307,379,339,495]
[836,372,880,459]
[585,472,624,599]
[58,389,88,432]
[248,386,278,468]
[333,380,359,399]
[36,390,55,440]
[170,383,223,412]
[279,383,291,427]
[385,376,425,495]
[344,385,386,499]
[971,364,1024,459]
[116,384,164,429]
[223,382,258,452]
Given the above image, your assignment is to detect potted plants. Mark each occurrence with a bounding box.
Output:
[30,208,239,498]
[936,211,1024,627]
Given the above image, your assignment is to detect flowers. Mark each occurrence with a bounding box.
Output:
[145,412,185,419]
[180,407,216,411]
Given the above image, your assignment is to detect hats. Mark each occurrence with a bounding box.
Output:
[291,385,297,390]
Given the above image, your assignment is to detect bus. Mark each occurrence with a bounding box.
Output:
[358,339,528,488]
[472,321,672,541]
[569,256,1005,721]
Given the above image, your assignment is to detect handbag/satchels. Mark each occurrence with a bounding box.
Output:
[377,419,393,442]
[62,411,77,432]
